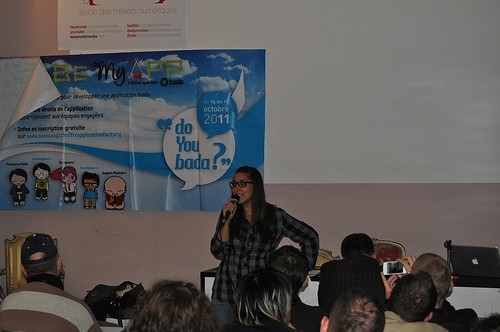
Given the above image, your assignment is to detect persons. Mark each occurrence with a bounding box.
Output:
[1,165,499,332]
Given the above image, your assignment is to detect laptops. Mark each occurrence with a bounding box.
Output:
[449,245,500,278]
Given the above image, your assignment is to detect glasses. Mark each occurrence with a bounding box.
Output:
[229,181,254,188]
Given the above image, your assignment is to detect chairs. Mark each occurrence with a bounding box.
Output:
[315,249,341,267]
[372,238,407,266]
[0,232,65,301]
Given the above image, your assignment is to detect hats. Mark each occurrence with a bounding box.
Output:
[20,232,59,266]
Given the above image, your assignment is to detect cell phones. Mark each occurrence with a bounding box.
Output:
[383,260,406,274]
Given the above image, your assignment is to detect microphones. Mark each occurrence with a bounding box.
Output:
[220,194,240,228]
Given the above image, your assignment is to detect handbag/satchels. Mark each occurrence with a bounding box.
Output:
[81,280,143,322]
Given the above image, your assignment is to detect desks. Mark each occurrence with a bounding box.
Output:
[199,267,500,332]
